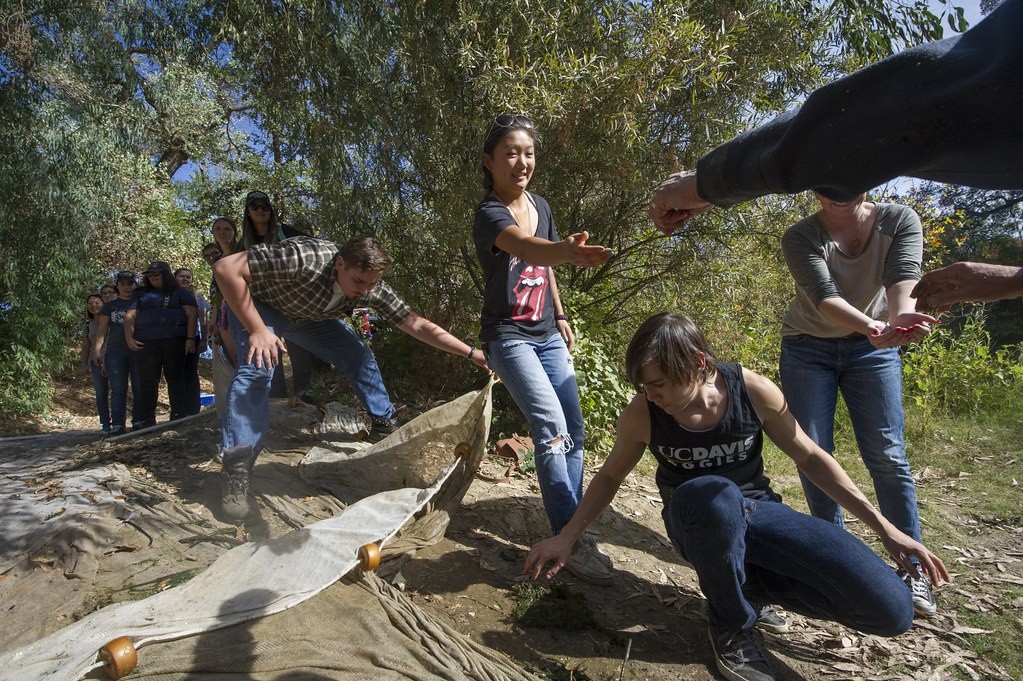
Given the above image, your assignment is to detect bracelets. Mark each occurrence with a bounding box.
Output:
[467,346,478,360]
[554,315,569,320]
[187,337,195,341]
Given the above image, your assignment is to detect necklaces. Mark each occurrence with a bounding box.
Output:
[495,192,533,237]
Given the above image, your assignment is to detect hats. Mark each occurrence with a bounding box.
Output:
[142,262,170,275]
[246,190,270,205]
[118,271,134,282]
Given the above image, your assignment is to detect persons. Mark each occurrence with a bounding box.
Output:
[202,191,316,465]
[521,311,951,681]
[646,0,1022,312]
[81,262,208,436]
[210,235,498,526]
[778,188,938,617]
[471,111,614,587]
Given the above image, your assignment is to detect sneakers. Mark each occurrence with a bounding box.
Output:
[708,620,774,681]
[898,563,937,614]
[221,447,263,527]
[555,536,615,585]
[740,583,788,632]
[366,405,407,442]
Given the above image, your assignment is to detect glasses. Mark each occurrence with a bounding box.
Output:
[249,204,271,211]
[101,290,117,296]
[484,115,534,147]
[204,251,222,261]
[147,273,161,277]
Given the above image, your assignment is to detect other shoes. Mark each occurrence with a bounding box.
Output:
[134,421,152,433]
[100,425,109,436]
[112,425,123,435]
